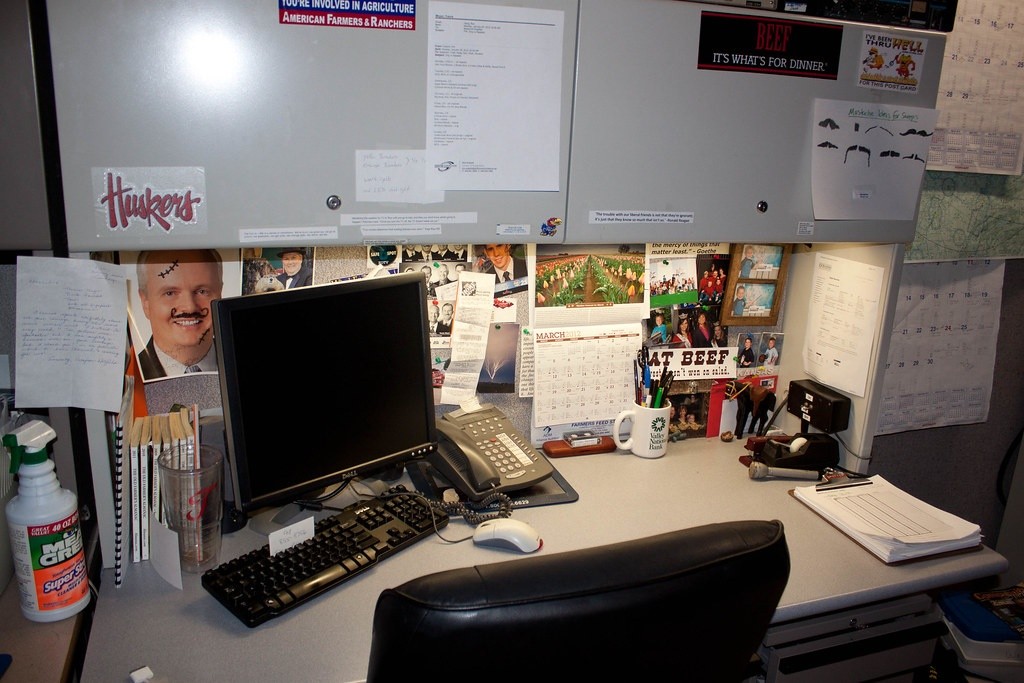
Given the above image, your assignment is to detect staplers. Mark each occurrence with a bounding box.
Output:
[739,436,794,467]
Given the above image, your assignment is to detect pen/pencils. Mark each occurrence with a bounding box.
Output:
[633,346,674,408]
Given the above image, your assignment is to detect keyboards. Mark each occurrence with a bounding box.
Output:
[201,484,449,628]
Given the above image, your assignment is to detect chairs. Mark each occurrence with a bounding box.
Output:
[367,518,790,683]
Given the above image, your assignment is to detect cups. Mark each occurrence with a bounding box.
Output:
[613,397,671,459]
[157,444,224,574]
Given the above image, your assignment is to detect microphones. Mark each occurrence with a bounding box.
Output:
[749,462,822,482]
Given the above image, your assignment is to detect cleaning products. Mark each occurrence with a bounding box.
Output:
[2,419,91,623]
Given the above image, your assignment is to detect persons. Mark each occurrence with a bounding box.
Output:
[731,286,754,316]
[429,302,453,337]
[671,397,695,425]
[698,264,725,306]
[138,249,224,380]
[651,314,667,344]
[672,312,725,348]
[431,263,466,296]
[402,244,467,261]
[484,244,528,284]
[738,338,755,368]
[404,266,437,297]
[740,247,759,277]
[267,248,312,291]
[763,338,778,366]
[650,275,694,297]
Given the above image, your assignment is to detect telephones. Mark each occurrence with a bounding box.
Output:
[430,402,553,500]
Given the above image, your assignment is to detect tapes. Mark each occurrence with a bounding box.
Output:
[789,437,807,453]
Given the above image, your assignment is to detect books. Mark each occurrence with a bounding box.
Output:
[112,373,203,588]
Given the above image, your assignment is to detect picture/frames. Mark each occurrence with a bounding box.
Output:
[720,243,792,327]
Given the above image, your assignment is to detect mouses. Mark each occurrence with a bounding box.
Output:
[473,518,543,553]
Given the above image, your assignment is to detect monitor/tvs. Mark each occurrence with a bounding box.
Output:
[210,272,438,537]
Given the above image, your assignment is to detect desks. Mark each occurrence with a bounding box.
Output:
[74,428,1003,683]
